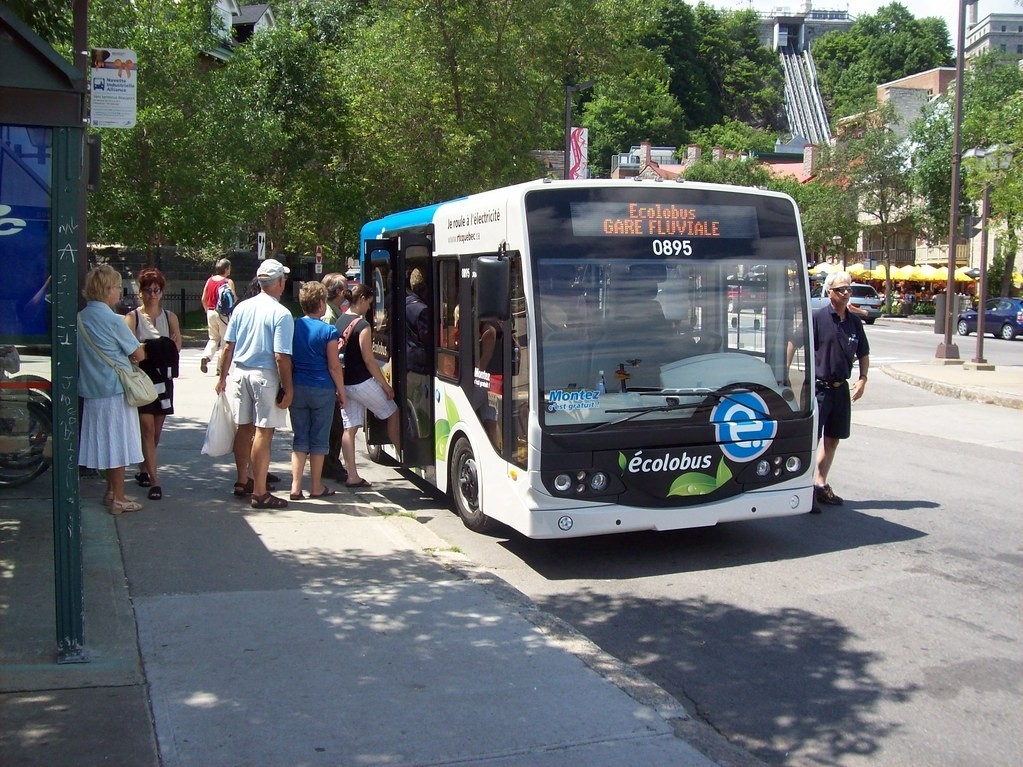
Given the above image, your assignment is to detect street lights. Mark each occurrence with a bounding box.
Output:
[960,140,1013,365]
[563,78,599,179]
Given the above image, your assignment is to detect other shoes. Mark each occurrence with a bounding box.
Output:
[217,372,229,376]
[200,358,208,373]
[267,472,280,482]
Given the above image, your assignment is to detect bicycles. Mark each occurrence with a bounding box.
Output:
[0,345,53,489]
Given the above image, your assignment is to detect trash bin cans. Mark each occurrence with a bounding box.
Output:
[901,303,912,315]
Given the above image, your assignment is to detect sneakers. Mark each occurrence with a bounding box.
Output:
[816,483,843,505]
[809,485,821,514]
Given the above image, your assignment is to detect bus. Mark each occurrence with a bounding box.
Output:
[357,179,819,541]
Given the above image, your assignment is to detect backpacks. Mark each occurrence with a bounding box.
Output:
[215,278,235,314]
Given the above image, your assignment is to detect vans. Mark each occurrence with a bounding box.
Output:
[810,284,881,324]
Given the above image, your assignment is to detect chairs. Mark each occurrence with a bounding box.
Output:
[541,330,589,389]
[592,332,652,390]
[616,277,668,329]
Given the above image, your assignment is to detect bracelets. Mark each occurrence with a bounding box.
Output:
[859,374,867,380]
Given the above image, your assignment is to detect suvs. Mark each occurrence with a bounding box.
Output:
[727,280,767,313]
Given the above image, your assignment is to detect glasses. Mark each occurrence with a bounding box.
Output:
[359,288,374,298]
[143,287,162,294]
[829,286,853,294]
[110,284,123,290]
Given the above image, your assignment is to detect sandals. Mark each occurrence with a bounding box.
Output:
[110,496,143,514]
[251,492,288,508]
[102,493,138,506]
[234,477,275,496]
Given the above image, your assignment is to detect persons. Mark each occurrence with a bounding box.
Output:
[288,280,345,502]
[201,259,237,375]
[405,267,436,373]
[125,269,182,500]
[215,258,295,508]
[787,271,870,514]
[320,273,349,483]
[847,301,868,317]
[511,265,588,340]
[336,285,399,488]
[466,319,521,452]
[875,285,972,307]
[444,303,459,378]
[76,265,146,515]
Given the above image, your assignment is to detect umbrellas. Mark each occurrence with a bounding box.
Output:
[787,262,977,283]
[1011,273,1023,282]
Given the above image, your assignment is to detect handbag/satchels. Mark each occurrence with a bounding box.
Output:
[338,318,363,368]
[119,363,158,407]
[201,391,237,457]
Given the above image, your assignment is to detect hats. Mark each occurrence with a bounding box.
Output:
[257,259,290,281]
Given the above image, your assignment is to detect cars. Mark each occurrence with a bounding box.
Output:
[956,296,1023,341]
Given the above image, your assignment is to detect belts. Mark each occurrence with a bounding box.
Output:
[815,379,846,387]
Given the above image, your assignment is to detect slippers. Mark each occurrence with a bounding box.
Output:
[345,477,372,487]
[290,490,305,499]
[135,472,151,487]
[310,486,335,498]
[148,486,162,500]
